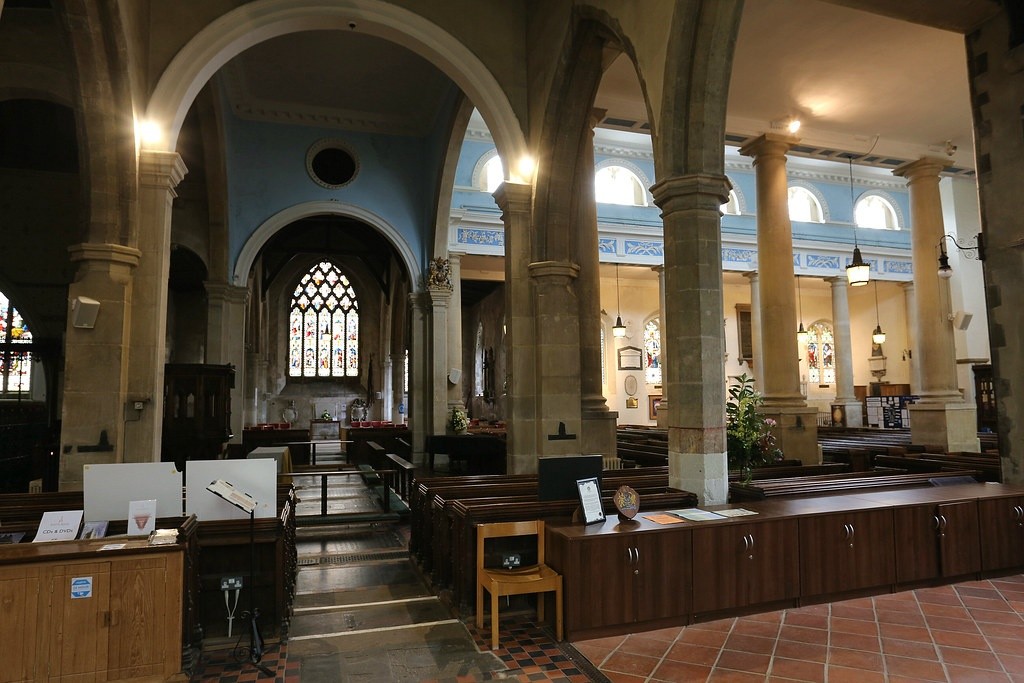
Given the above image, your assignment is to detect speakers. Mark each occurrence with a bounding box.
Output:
[449,368,462,385]
[73,296,100,328]
[954,311,974,330]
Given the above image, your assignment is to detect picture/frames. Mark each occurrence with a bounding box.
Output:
[648,394,664,419]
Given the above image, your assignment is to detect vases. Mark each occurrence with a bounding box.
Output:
[449,427,467,435]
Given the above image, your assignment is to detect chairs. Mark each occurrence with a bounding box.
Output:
[245,422,292,430]
[477,520,564,650]
[350,421,408,427]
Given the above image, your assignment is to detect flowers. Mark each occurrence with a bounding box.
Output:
[445,406,471,430]
[725,372,785,488]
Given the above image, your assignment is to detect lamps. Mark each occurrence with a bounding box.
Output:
[946,310,974,331]
[612,263,626,339]
[872,280,886,344]
[797,274,808,343]
[72,297,100,329]
[845,154,871,288]
[205,478,282,677]
[935,233,986,279]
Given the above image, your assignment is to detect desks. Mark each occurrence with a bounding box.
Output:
[425,434,500,475]
[341,427,412,462]
[309,420,342,439]
[243,430,310,482]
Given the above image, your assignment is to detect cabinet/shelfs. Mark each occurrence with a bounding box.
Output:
[879,384,910,396]
[546,484,1024,644]
[0,515,199,683]
[854,386,867,400]
[972,364,997,406]
